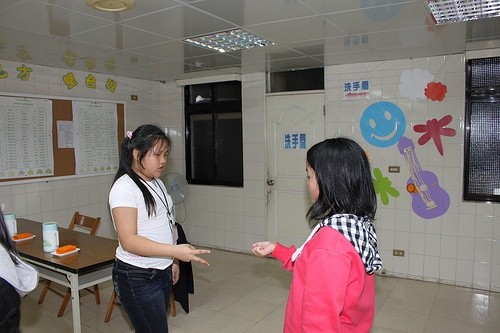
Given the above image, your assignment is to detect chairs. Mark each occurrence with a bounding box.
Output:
[38,212,179,323]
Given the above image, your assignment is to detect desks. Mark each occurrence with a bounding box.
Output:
[14,217,120,333]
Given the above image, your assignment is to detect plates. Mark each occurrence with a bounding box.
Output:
[51,248,80,257]
[11,235,35,242]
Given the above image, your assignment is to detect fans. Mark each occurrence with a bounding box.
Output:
[161,172,189,222]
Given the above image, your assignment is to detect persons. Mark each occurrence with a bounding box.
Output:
[251,137,382,333]
[0,208,38,333]
[108,124,211,333]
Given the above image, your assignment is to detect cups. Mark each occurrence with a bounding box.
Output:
[43,222,59,252]
[3,213,17,237]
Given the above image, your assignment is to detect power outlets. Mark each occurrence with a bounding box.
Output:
[393,249,404,256]
[389,166,400,173]
